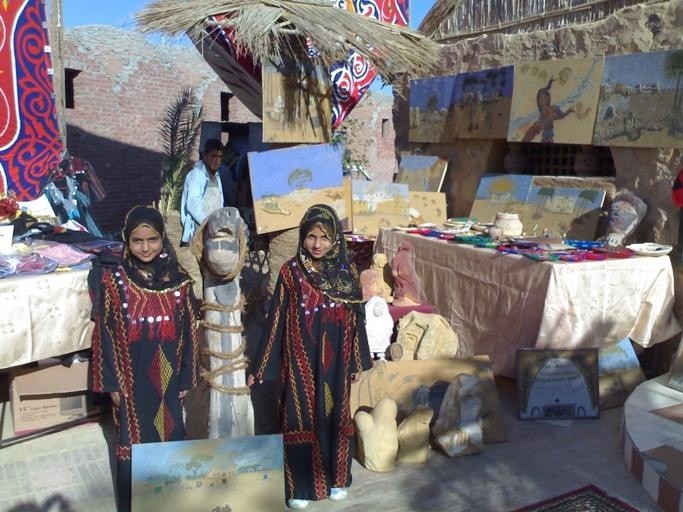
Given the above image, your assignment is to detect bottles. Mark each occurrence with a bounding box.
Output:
[493,213,523,237]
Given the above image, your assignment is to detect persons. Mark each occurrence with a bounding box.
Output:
[180,138,224,245]
[244,203,374,510]
[89,204,199,512]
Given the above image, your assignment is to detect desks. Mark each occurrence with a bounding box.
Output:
[0,196,125,450]
[372,222,682,383]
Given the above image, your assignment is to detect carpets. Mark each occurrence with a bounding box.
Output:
[510,483,640,512]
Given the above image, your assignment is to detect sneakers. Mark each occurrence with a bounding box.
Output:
[328,488,348,501]
[287,498,308,509]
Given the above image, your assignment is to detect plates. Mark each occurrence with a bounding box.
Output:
[420,217,477,234]
[625,241,674,258]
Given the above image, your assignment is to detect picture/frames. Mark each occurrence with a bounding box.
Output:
[515,348,600,421]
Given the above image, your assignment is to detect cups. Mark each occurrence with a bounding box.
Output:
[490,227,502,238]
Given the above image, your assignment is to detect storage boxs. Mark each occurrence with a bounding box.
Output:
[8,354,103,436]
